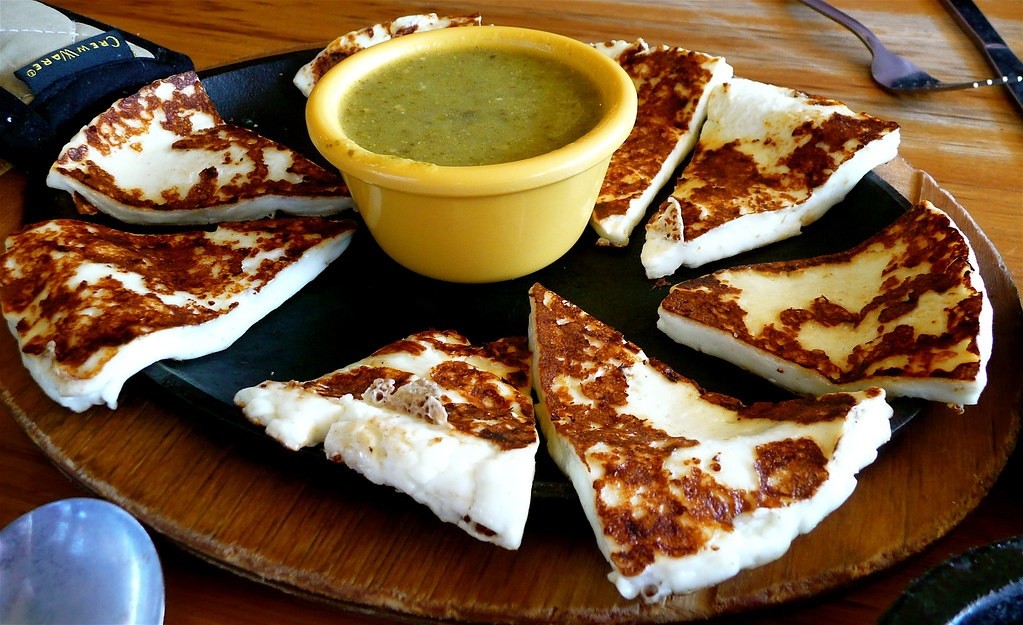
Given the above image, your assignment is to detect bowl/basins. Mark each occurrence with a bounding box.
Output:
[304,25,638,284]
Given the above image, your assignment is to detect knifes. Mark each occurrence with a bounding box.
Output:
[938,0,1023,112]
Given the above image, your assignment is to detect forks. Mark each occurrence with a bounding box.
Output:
[801,0,1023,96]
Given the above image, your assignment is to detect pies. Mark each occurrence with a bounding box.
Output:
[0,13,994,602]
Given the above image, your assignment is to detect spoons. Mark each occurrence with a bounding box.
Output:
[0,496,165,625]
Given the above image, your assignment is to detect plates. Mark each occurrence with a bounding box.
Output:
[22,48,941,499]
